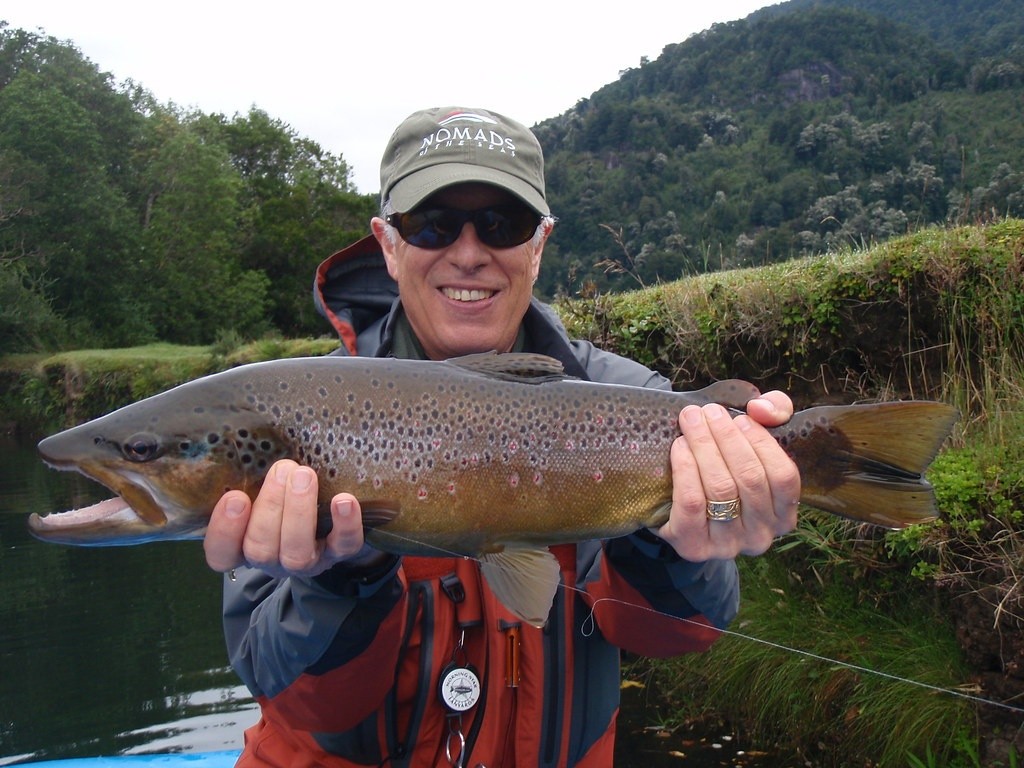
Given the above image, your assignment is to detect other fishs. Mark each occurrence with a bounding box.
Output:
[28,350,962,546]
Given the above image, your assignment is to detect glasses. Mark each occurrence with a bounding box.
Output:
[385,194,541,249]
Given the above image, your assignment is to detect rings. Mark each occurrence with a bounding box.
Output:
[706,497,741,521]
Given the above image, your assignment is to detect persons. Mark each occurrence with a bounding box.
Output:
[202,105,801,768]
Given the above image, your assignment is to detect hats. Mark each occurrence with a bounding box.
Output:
[379,105,550,220]
